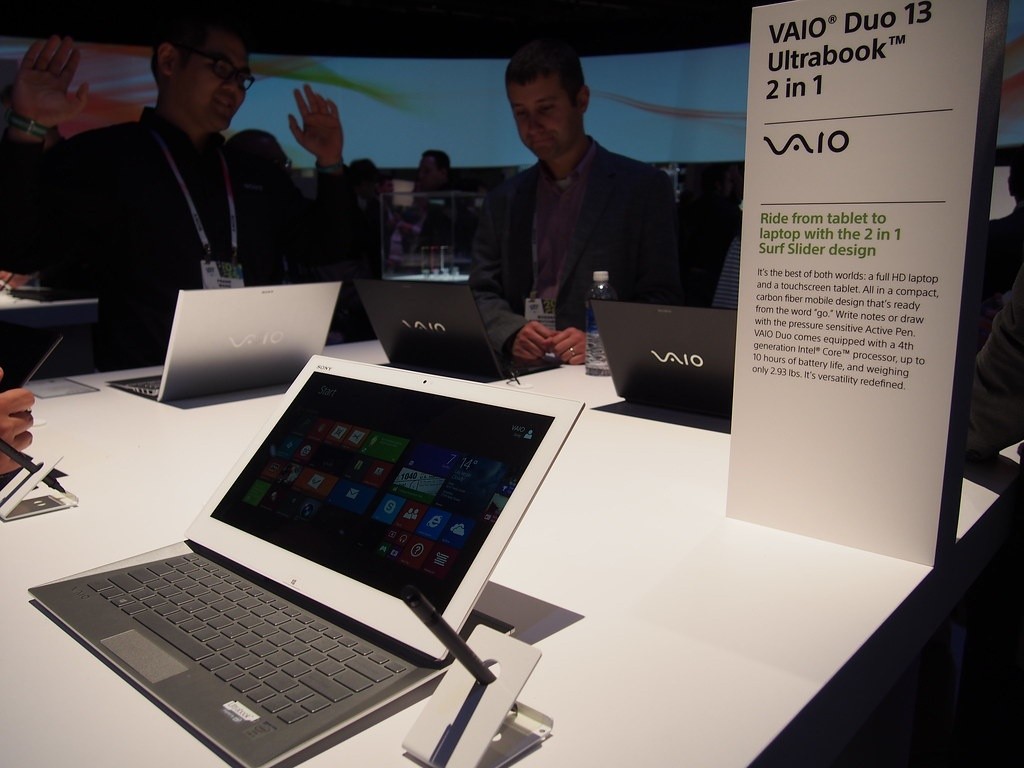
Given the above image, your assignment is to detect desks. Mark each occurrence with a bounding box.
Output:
[0,336,1024,768]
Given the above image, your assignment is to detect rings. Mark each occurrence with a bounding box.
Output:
[569,346,578,356]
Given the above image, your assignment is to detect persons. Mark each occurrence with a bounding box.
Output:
[223,129,478,286]
[0,19,342,371]
[677,163,742,307]
[966,143,1024,464]
[469,39,683,366]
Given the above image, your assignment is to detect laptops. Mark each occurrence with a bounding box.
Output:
[26,352,586,768]
[105,280,342,402]
[355,275,563,381]
[591,297,738,420]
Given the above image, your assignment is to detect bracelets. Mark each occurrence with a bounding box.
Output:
[312,156,344,174]
[5,107,49,138]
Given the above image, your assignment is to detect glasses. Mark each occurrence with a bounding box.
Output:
[189,47,256,91]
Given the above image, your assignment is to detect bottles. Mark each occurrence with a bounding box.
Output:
[586,271,615,375]
